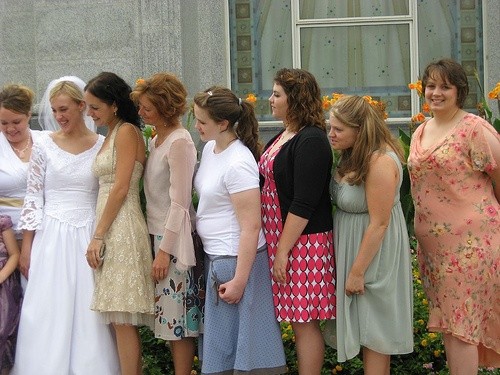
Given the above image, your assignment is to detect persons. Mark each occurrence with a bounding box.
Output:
[19,76,121,375]
[324,94,415,375]
[257,67,337,375]
[128,71,208,375]
[192,85,288,375]
[406,56,500,375]
[0,83,42,240]
[0,214,23,375]
[83,72,156,375]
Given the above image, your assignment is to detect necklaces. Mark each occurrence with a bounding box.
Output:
[156,134,161,147]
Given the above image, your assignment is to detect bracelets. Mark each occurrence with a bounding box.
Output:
[92,235,105,240]
